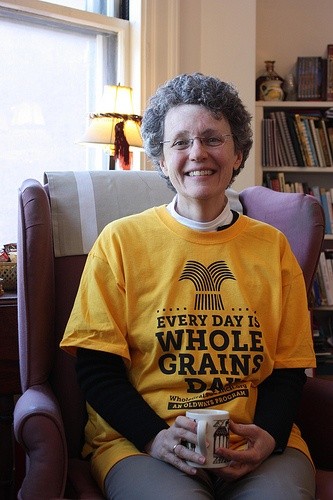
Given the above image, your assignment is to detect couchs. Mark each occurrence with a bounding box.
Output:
[14,170,333,500]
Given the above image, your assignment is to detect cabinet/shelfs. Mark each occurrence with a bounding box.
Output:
[256,100,333,364]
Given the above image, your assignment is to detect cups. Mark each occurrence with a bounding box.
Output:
[186,409,230,468]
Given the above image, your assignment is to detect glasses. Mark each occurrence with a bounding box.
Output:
[158,133,234,148]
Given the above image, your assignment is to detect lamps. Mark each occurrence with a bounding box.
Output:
[86,85,146,170]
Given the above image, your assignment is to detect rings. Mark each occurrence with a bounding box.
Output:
[172,443,180,454]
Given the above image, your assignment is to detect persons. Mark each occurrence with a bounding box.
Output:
[60,72,317,500]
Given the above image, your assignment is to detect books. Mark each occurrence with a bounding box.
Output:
[326,44,333,101]
[262,107,333,168]
[315,250,333,306]
[262,172,333,234]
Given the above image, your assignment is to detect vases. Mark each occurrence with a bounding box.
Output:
[256,61,286,101]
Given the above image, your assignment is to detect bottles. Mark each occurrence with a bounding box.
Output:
[283,74,298,101]
[255,60,286,102]
